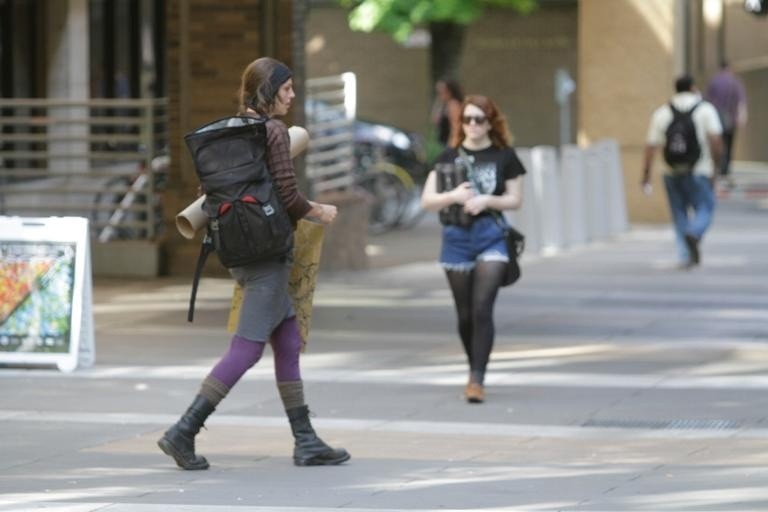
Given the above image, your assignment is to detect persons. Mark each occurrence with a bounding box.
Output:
[155,57,353,472]
[640,72,732,274]
[418,93,527,404]
[430,74,465,152]
[701,58,749,190]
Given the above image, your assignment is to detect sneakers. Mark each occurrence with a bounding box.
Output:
[682,232,703,263]
[466,379,489,402]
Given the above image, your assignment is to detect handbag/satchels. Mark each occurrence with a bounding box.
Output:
[501,227,525,285]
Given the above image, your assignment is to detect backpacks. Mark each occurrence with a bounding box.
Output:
[184,114,295,267]
[663,103,701,168]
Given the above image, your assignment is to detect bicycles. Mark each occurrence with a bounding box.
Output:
[345,155,426,231]
[92,132,172,244]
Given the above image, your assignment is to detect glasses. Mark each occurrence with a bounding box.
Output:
[462,116,486,123]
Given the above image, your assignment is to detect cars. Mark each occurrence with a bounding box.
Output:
[304,98,429,237]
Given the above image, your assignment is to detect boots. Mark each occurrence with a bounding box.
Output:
[286,405,350,466]
[158,394,216,471]
